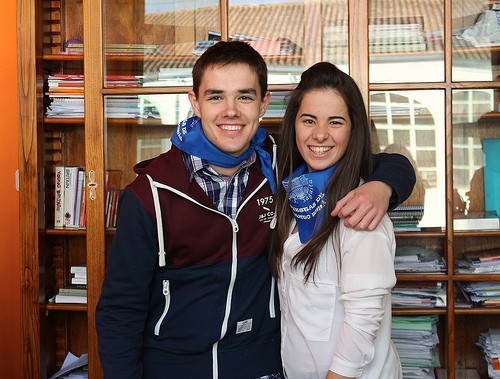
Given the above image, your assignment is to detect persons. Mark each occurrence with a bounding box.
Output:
[96,40,416,379]
[368,111,500,218]
[269,62,405,379]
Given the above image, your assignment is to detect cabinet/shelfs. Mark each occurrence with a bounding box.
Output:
[15,0,500,379]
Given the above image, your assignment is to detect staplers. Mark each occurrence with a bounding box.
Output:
[205,29,270,43]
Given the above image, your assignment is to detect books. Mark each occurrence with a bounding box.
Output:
[54,265,87,303]
[388,205,424,232]
[370,105,420,116]
[262,91,291,117]
[323,0,500,53]
[441,211,499,233]
[192,30,302,55]
[58,43,165,55]
[54,167,123,228]
[47,67,191,88]
